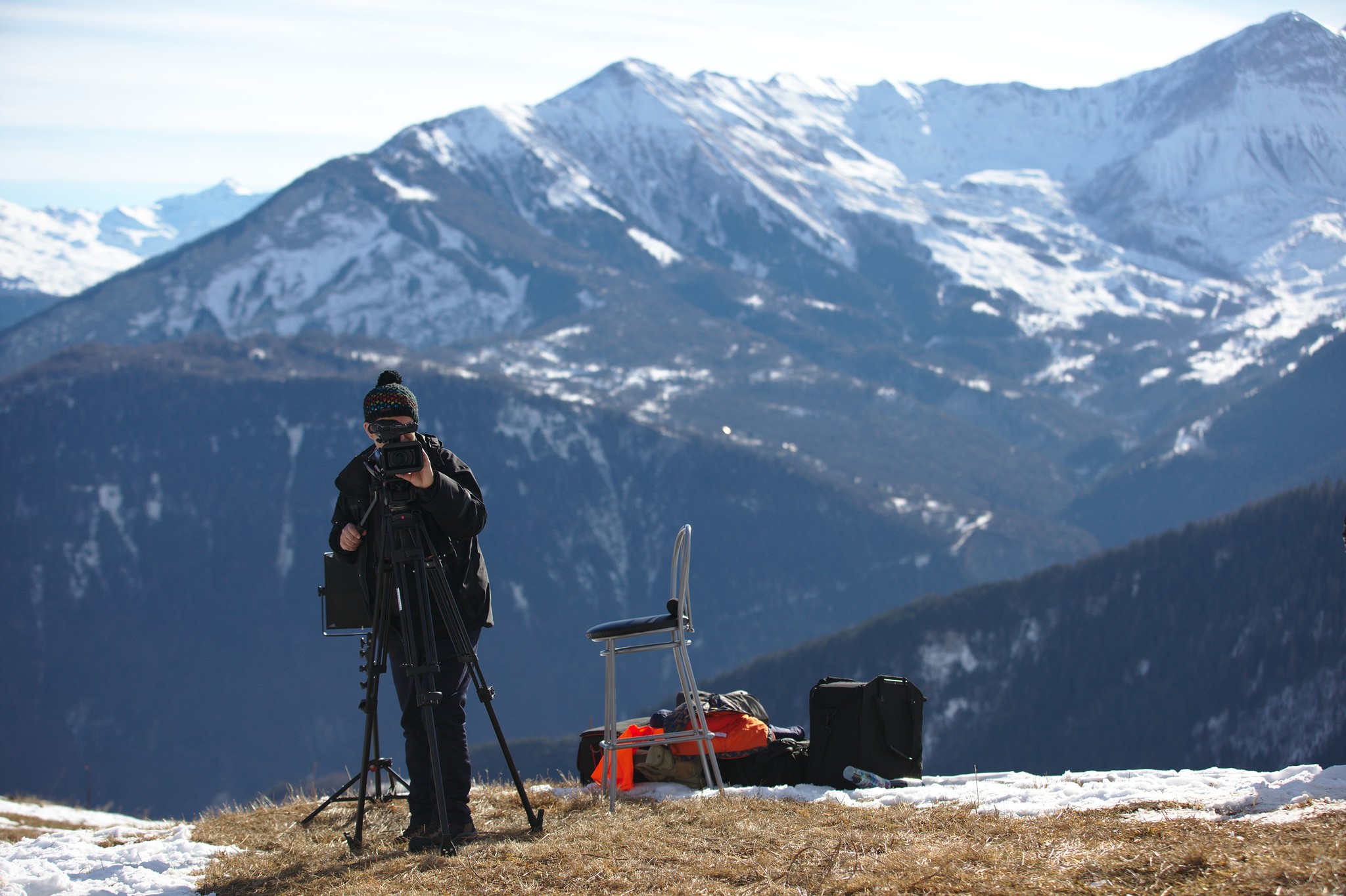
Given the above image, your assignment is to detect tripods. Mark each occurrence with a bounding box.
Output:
[343,481,545,848]
[297,631,410,826]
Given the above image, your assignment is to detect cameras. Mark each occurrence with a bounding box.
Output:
[364,422,424,481]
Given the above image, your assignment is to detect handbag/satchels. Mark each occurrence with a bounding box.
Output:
[808,675,927,790]
[577,716,652,785]
[634,745,703,789]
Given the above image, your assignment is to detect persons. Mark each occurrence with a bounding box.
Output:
[327,371,495,850]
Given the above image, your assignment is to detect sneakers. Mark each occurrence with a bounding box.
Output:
[393,823,429,845]
[408,818,479,854]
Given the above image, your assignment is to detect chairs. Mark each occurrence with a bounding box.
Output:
[582,522,727,816]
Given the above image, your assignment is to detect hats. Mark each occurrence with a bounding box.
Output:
[364,370,419,424]
